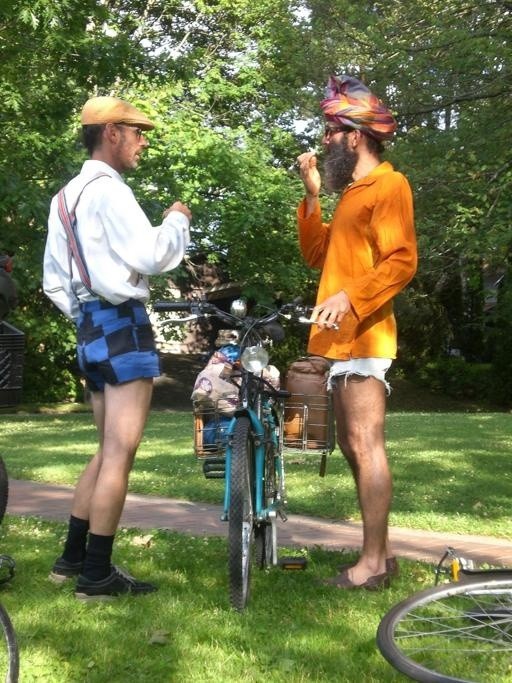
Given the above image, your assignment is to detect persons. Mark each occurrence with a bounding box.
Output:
[38,93,196,600]
[292,70,420,594]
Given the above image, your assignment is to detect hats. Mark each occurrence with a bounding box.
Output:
[79,95,156,131]
[319,74,397,143]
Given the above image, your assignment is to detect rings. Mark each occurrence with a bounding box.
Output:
[293,163,300,169]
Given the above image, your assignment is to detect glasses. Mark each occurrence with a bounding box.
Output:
[116,123,142,141]
[324,124,355,141]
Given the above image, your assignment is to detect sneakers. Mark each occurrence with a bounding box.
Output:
[314,556,402,593]
[46,556,159,602]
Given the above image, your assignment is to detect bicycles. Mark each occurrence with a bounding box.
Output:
[151,289,341,610]
[375,548,511,682]
